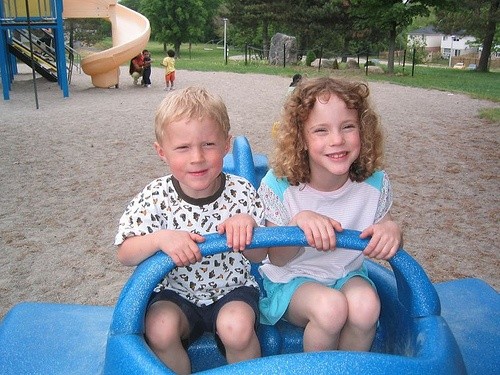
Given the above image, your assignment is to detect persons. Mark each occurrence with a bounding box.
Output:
[129,50,154,87]
[257,77,404,353]
[114,87,269,375]
[287,73,302,97]
[160,49,176,91]
[37,28,55,57]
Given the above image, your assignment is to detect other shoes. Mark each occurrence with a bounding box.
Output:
[133,81,137,86]
[164,87,175,91]
[141,84,150,87]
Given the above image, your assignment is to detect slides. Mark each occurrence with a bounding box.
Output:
[79,3,151,90]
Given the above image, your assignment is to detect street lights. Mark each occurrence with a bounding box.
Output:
[222,18,228,64]
